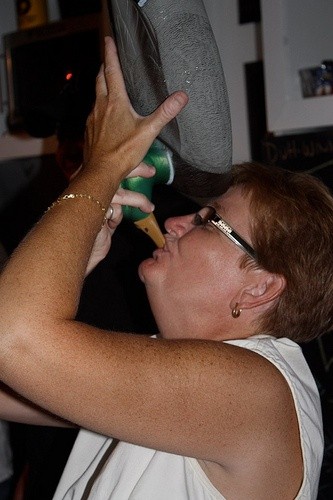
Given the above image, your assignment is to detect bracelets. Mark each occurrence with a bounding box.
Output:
[43,193,108,234]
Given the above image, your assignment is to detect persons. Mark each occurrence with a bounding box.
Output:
[0,32,333,500]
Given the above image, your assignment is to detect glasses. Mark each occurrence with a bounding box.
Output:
[191,207,278,275]
